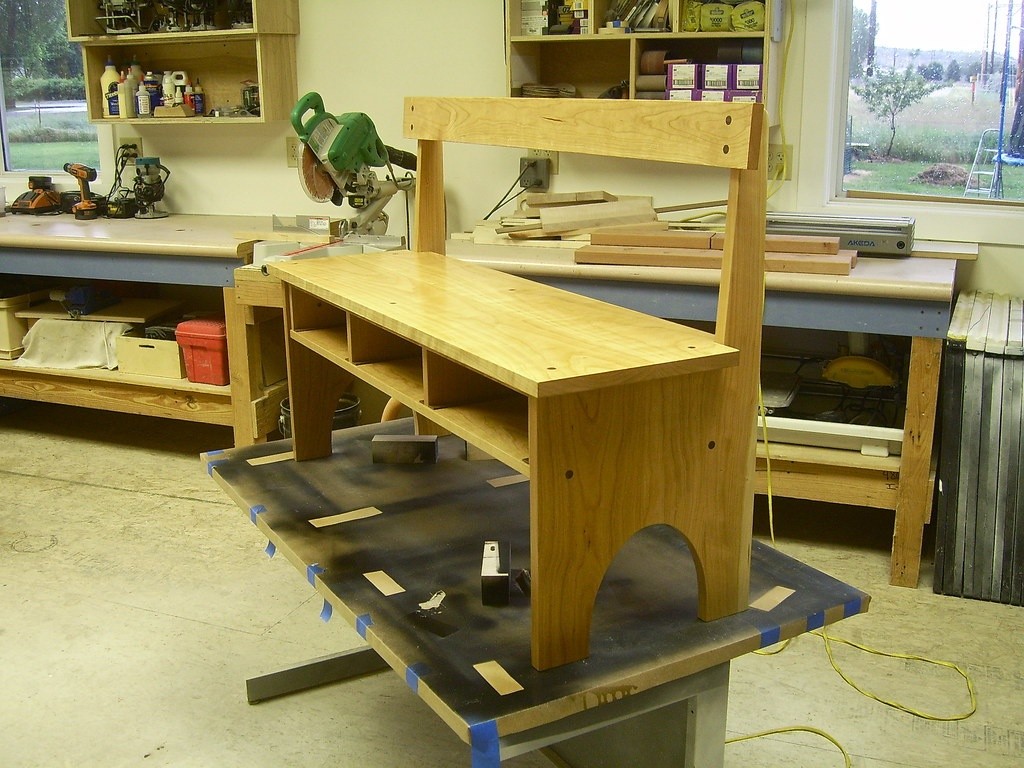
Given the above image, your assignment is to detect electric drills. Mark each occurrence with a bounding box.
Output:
[63,163,98,220]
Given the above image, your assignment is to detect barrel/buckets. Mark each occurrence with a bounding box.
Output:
[278,393,363,439]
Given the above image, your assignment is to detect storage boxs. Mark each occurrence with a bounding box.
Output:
[521,0,555,36]
[733,64,762,90]
[702,64,733,90]
[729,90,761,103]
[0,283,64,360]
[175,310,231,387]
[665,89,697,101]
[116,324,188,379]
[697,90,730,102]
[667,64,702,90]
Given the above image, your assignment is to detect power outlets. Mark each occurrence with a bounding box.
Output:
[119,137,144,166]
[519,157,550,188]
[286,136,299,168]
[528,148,558,175]
[767,143,794,181]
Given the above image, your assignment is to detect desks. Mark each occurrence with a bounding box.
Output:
[203,414,872,768]
[0,214,957,589]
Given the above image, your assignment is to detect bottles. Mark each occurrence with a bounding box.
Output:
[99,51,204,119]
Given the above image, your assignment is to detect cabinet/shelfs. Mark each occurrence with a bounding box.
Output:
[503,0,784,128]
[65,0,300,124]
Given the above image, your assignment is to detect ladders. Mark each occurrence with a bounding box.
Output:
[963,129,1005,199]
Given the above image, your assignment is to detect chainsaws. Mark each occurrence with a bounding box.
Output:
[255,91,416,266]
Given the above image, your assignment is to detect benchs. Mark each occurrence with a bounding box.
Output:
[266,94,771,672]
